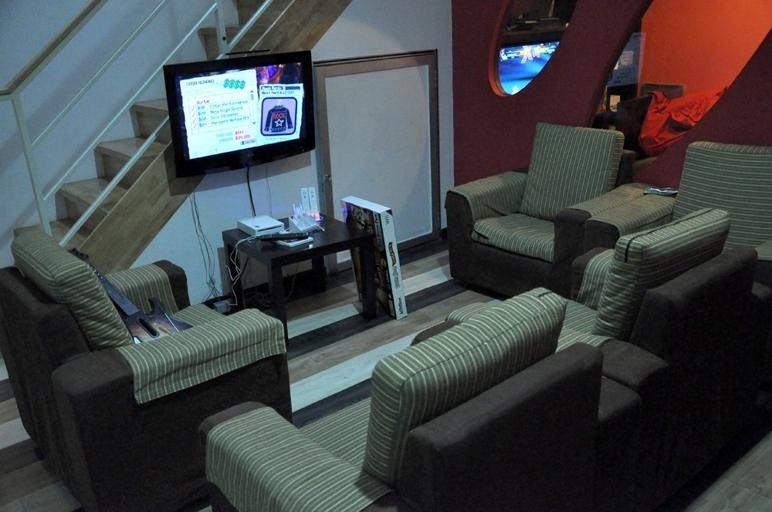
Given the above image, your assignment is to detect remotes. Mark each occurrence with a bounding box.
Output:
[260,232,309,241]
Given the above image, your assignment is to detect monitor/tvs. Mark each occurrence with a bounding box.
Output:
[497,27,566,96]
[163,50,315,178]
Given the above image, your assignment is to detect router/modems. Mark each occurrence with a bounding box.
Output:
[237,215,284,236]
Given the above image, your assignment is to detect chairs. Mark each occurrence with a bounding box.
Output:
[196,286,645,509]
[443,122,636,302]
[582,139,772,259]
[446,205,771,485]
[0,223,293,509]
